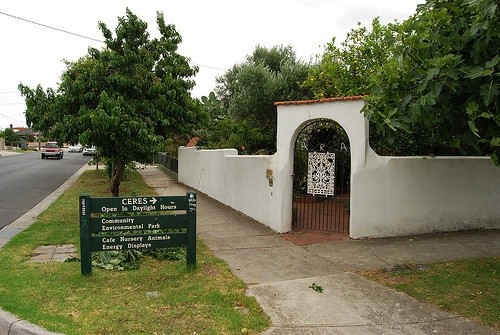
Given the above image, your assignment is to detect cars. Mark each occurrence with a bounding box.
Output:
[68,143,83,152]
[41,142,64,159]
[83,145,97,156]
